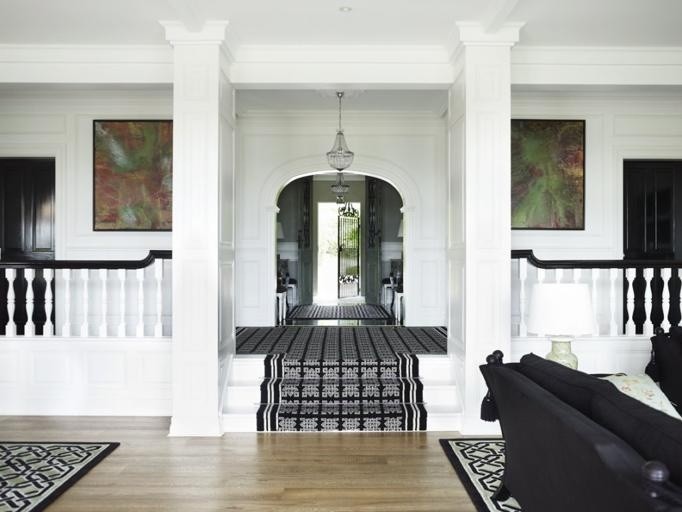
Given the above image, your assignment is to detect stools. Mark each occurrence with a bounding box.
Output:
[278,279,297,323]
[381,278,403,323]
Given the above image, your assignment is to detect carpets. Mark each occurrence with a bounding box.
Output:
[286,303,388,319]
[440,436,523,512]
[0,441,122,511]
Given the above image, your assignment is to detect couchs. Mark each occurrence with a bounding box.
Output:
[481,348,681,511]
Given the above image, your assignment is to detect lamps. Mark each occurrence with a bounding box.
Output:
[324,92,355,202]
[528,283,597,376]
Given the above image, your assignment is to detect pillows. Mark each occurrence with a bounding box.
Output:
[604,369,680,420]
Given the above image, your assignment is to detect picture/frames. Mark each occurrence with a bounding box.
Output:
[92,118,173,232]
[509,118,585,229]
